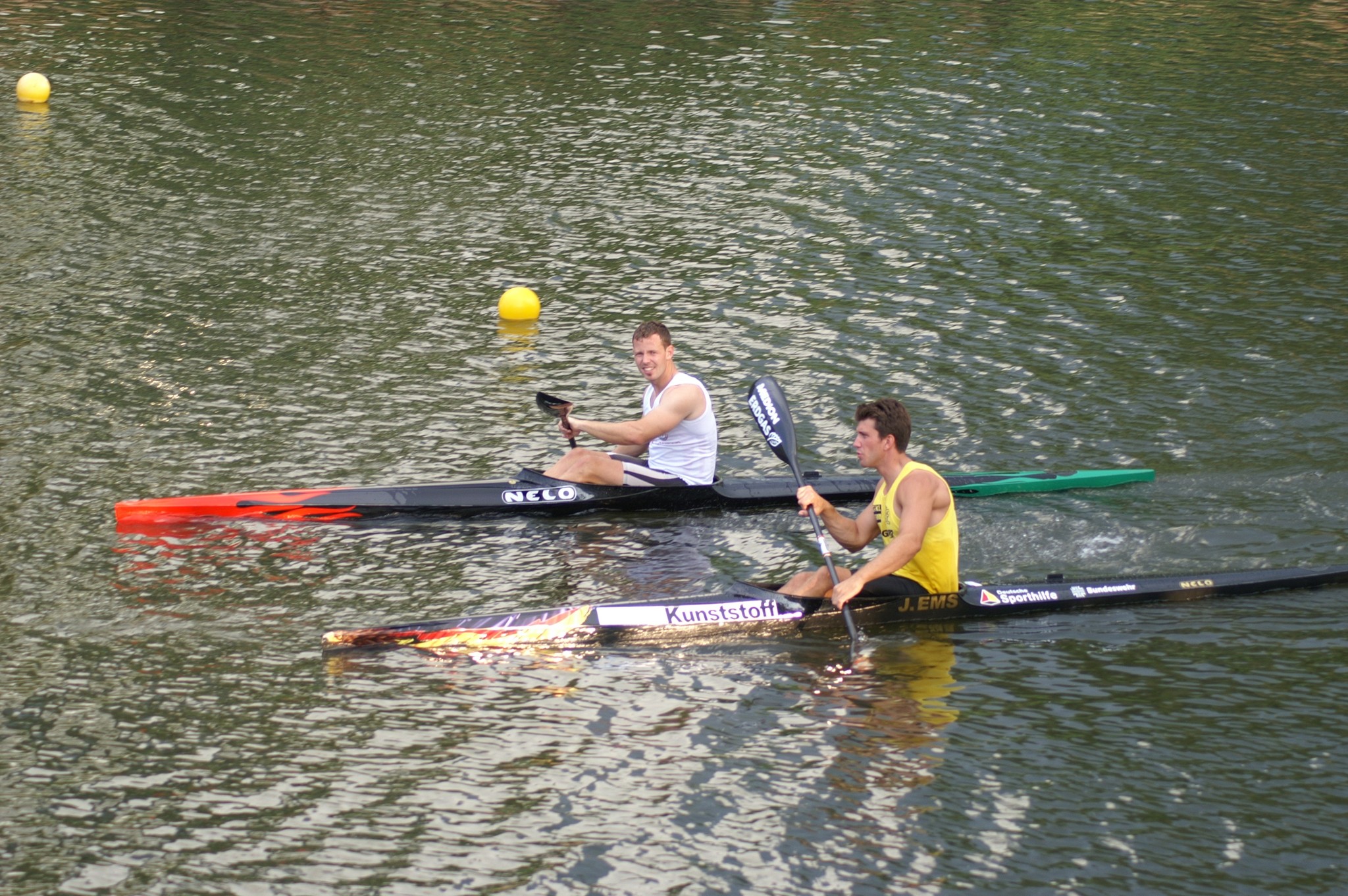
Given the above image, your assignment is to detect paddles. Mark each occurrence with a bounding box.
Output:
[746,374,865,660]
[535,390,578,450]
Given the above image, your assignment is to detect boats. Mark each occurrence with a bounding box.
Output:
[113,468,1158,523]
[320,564,1348,648]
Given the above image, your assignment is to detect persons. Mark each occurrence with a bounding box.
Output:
[777,398,958,609]
[541,323,718,488]
